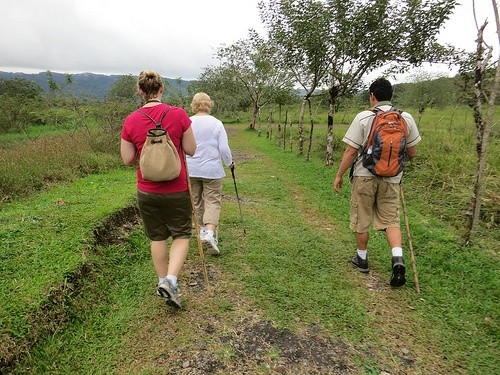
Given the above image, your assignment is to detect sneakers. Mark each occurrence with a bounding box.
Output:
[349,255,369,273]
[158,279,180,307]
[389,256,407,287]
[203,235,221,255]
[199,229,219,246]
[158,284,181,296]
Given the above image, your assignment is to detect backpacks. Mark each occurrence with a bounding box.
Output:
[349,106,410,179]
[135,106,182,182]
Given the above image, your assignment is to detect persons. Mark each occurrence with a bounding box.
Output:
[333,78,421,288]
[120,70,197,310]
[186,92,235,255]
